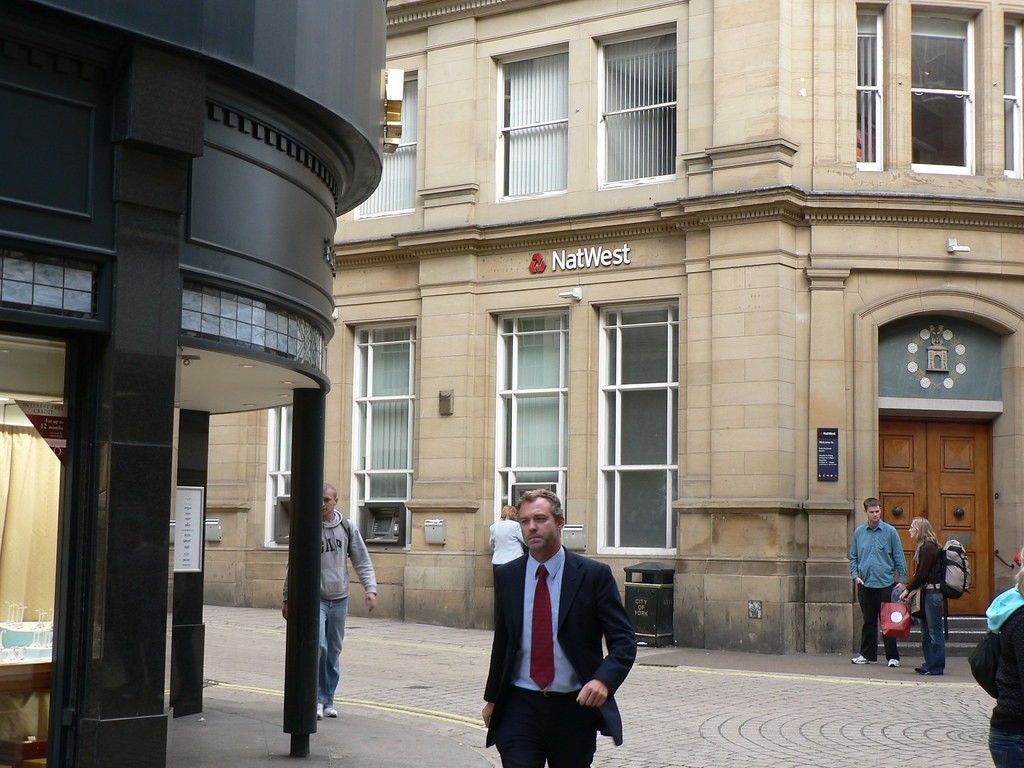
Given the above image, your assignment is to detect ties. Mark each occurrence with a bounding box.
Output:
[530,566,555,689]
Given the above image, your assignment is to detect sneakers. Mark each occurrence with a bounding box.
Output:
[851,655,877,664]
[323,708,337,717]
[888,659,899,667]
[317,704,323,718]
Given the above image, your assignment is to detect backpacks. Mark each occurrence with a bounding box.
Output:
[941,539,971,600]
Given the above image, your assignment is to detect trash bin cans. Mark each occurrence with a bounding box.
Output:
[622,561,675,647]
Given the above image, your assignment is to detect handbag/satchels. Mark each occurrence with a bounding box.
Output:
[968,632,999,699]
[880,602,912,639]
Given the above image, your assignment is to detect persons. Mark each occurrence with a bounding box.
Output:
[898,515,945,677]
[986,546,1024,768]
[850,497,908,667]
[480,489,638,768]
[487,503,528,571]
[281,482,378,720]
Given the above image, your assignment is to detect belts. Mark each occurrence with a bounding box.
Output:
[925,583,940,589]
